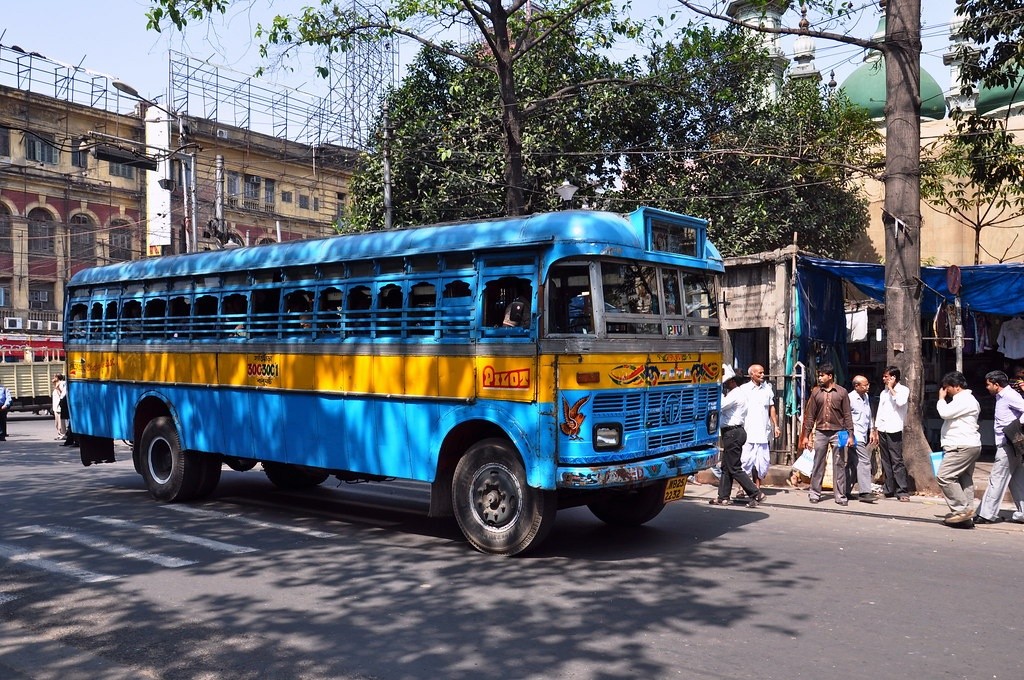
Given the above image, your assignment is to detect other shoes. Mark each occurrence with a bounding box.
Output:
[1004,518,1024,524]
[59,438,73,446]
[736,490,744,498]
[5,435,9,437]
[858,493,877,500]
[810,498,818,503]
[54,436,67,440]
[973,515,992,524]
[943,518,974,528]
[839,501,848,506]
[69,441,79,447]
[0,439,6,441]
[846,493,856,500]
[945,510,977,523]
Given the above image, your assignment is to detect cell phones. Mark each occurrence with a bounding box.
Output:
[890,375,896,382]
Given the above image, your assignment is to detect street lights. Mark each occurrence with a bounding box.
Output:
[111,79,191,254]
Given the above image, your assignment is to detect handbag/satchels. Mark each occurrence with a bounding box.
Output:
[791,449,816,478]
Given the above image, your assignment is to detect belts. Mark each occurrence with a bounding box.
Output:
[721,425,741,432]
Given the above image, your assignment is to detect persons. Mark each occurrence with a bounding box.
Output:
[937,371,982,528]
[709,362,767,508]
[504,278,531,329]
[0,379,12,441]
[845,375,877,502]
[973,369,1024,524]
[786,384,817,488]
[875,365,910,502]
[52,373,80,447]
[801,363,855,506]
[736,363,782,499]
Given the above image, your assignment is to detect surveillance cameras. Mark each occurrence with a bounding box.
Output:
[177,133,187,145]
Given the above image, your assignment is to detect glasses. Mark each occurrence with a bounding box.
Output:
[859,383,870,387]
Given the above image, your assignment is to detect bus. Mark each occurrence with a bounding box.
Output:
[62,206,731,559]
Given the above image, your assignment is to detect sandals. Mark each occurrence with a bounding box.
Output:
[709,498,728,506]
[897,493,910,502]
[745,493,767,508]
[877,494,886,499]
